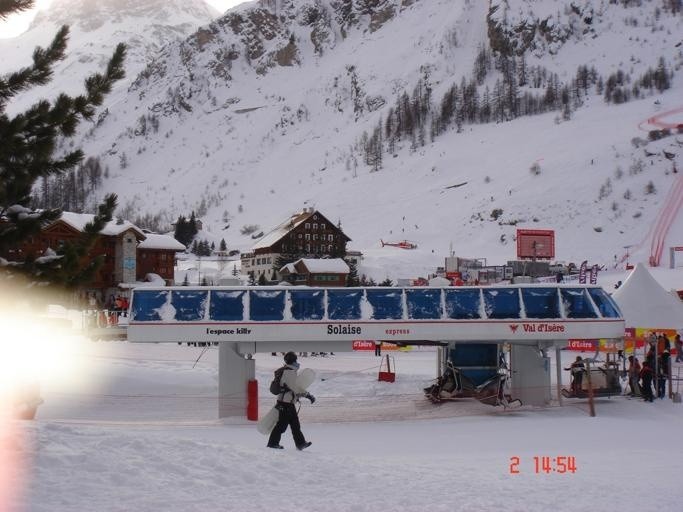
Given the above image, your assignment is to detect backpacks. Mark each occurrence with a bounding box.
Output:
[269,366,294,395]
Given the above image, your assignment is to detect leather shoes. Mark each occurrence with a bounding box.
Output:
[267,443,284,449]
[298,441,312,451]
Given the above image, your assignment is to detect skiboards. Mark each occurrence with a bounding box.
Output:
[622,393,644,400]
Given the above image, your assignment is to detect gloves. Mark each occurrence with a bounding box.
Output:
[306,393,315,404]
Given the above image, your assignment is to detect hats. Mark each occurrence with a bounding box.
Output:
[284,352,297,364]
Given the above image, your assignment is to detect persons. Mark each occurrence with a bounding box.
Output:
[628,333,682,402]
[564,355,583,391]
[374,340,383,357]
[268,351,315,450]
[78,291,128,318]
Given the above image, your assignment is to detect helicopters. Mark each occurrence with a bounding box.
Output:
[381,240,417,249]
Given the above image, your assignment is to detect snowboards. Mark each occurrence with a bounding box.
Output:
[256,369,316,435]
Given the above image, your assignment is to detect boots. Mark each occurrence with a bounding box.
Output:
[616,357,683,403]
